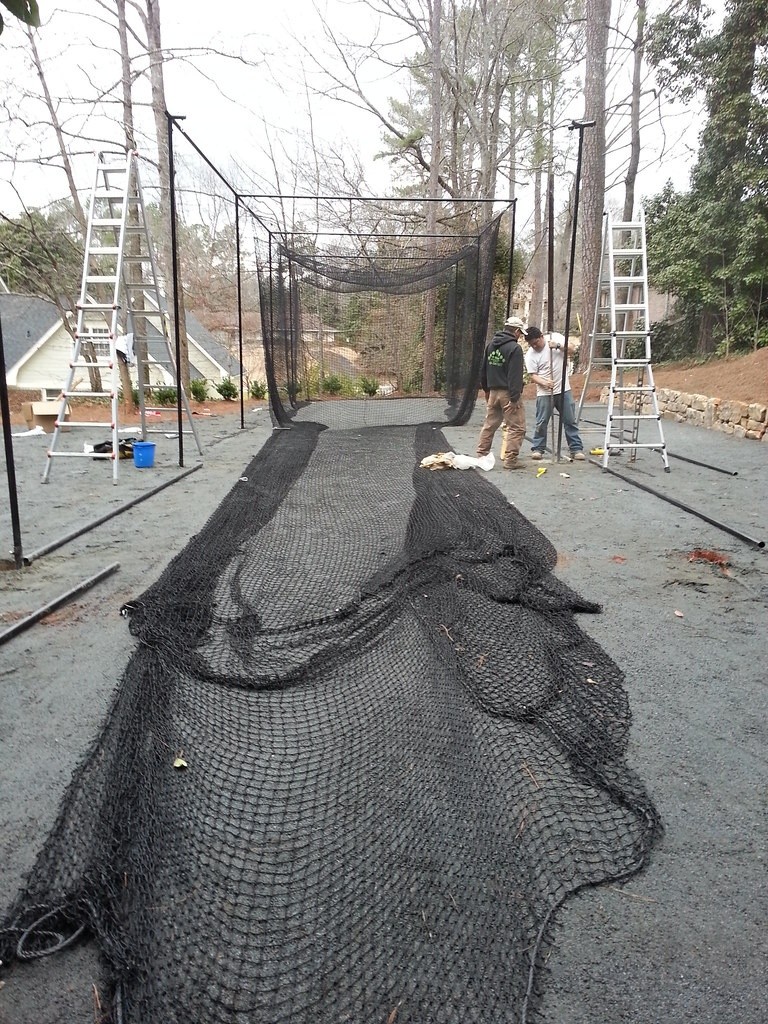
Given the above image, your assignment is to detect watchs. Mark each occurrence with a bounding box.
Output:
[556,342,561,349]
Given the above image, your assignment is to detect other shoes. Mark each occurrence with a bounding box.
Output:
[570,451,585,460]
[532,451,542,459]
[504,462,526,469]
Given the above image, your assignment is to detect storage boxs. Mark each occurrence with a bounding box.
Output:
[22,401,73,434]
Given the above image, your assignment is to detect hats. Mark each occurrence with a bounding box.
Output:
[505,317,528,336]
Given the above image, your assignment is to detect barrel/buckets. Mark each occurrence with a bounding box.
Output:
[132,442,156,468]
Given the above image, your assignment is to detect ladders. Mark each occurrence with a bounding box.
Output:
[41,150,205,484]
[577,209,671,475]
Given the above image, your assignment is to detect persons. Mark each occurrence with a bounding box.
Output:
[477,317,527,469]
[524,328,586,460]
